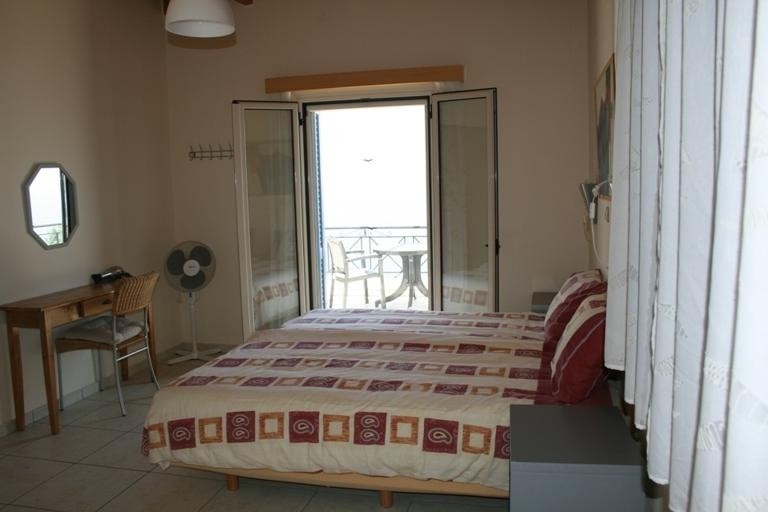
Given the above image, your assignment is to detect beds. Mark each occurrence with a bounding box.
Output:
[140,309,610,507]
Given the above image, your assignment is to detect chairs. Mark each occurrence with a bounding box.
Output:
[327,238,386,309]
[52,271,161,417]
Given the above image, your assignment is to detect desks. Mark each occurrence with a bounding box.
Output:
[0,279,157,436]
[373,245,428,308]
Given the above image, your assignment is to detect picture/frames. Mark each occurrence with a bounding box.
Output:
[593,54,615,200]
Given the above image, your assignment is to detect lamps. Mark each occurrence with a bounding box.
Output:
[164,0,237,38]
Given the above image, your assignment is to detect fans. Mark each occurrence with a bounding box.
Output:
[164,239,221,365]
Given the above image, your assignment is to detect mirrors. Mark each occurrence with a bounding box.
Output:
[23,162,79,251]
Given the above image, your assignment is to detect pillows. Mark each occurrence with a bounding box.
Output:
[544,269,603,336]
[547,282,609,405]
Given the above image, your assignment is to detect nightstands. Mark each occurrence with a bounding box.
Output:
[510,406,644,512]
[531,292,558,313]
